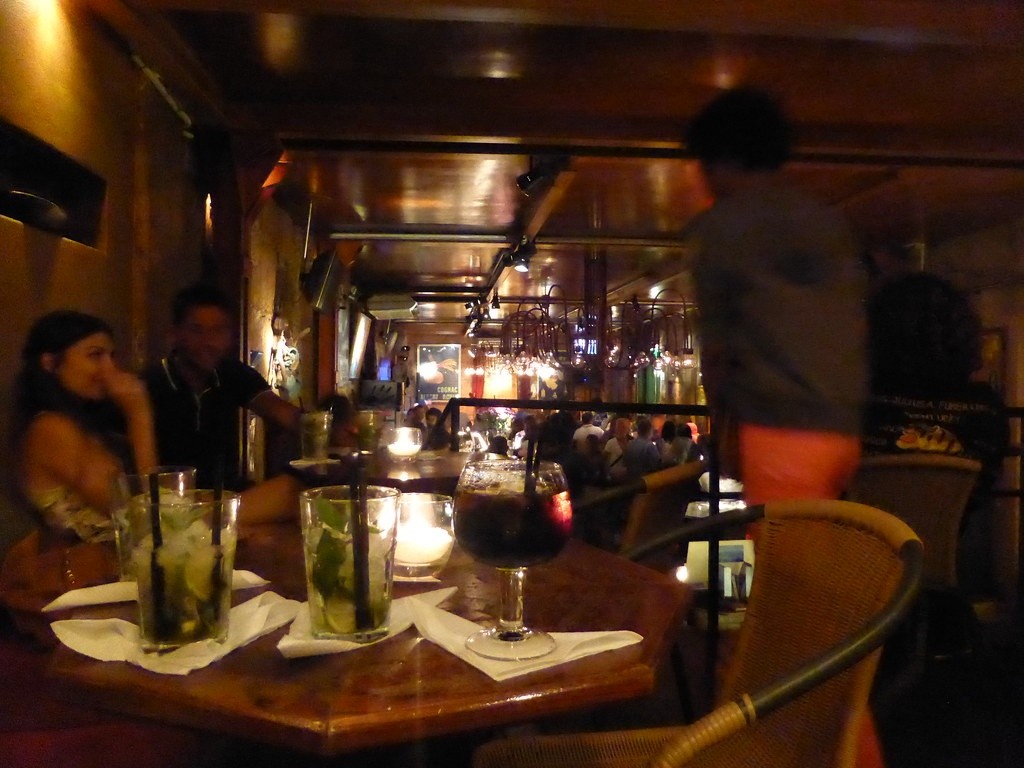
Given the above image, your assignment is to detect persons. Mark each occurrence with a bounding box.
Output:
[489,411,710,485]
[684,86,866,540]
[8,284,361,543]
[407,405,449,450]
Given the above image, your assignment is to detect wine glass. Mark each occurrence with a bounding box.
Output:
[450,459,575,661]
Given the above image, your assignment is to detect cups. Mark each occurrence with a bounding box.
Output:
[109,464,199,582]
[349,410,385,456]
[387,427,423,464]
[297,486,402,644]
[126,485,241,647]
[298,412,334,460]
[392,489,458,583]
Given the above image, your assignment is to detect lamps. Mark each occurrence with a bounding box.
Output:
[464,293,488,310]
[513,236,530,273]
[517,156,578,200]
[491,291,500,309]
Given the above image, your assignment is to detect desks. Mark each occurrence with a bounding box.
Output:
[40,443,692,768]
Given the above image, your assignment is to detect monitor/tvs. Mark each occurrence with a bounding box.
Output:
[307,250,343,315]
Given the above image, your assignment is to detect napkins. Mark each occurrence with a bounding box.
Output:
[392,573,441,585]
[276,586,460,659]
[404,595,645,684]
[40,568,271,612]
[49,589,302,675]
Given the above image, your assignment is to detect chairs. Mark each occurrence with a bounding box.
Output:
[466,444,1024,768]
